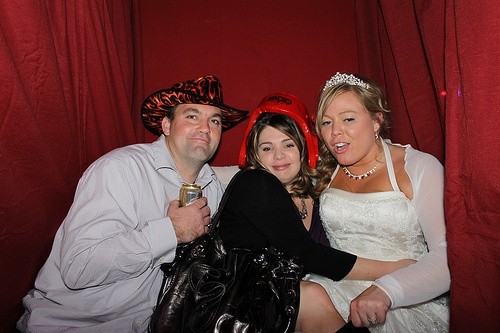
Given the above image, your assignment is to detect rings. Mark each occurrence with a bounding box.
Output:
[369,318,378,325]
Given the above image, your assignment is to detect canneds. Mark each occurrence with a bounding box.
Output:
[179,183,203,208]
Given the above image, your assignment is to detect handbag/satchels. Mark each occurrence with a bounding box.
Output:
[148,226,302,333]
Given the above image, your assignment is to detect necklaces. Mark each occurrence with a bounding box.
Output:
[299,197,308,220]
[343,151,385,180]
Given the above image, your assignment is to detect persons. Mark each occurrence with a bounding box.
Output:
[148,92,418,333]
[16,74,250,333]
[294,71,450,333]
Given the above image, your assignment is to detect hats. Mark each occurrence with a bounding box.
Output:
[140,74,249,136]
[238,92,318,169]
